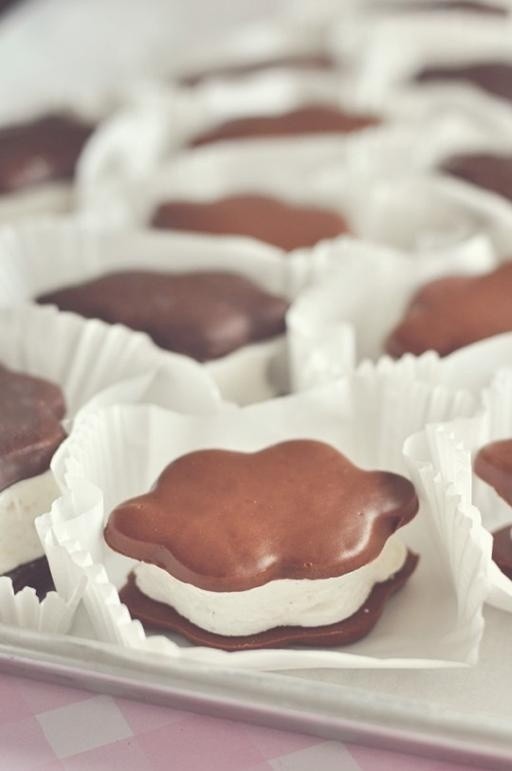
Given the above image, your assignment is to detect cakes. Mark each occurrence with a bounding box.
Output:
[100,437,424,653]
[404,52,511,108]
[171,98,381,150]
[171,41,336,94]
[382,253,512,362]
[31,260,294,368]
[428,144,511,225]
[142,187,352,256]
[467,432,512,618]
[1,360,70,495]
[1,104,99,203]
